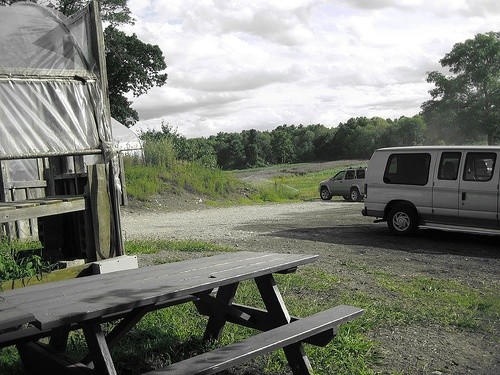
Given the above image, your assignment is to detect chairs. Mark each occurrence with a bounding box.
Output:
[445,161,457,179]
[474,160,491,181]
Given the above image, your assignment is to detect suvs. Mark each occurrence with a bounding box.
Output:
[318,166,367,203]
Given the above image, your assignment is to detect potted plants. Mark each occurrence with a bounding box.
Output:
[15,240,44,259]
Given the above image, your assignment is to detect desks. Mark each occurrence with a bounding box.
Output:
[0,249,315,375]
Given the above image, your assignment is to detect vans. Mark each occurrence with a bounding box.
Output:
[362,146,499,245]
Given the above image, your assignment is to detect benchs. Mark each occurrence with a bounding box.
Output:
[140,305,365,374]
